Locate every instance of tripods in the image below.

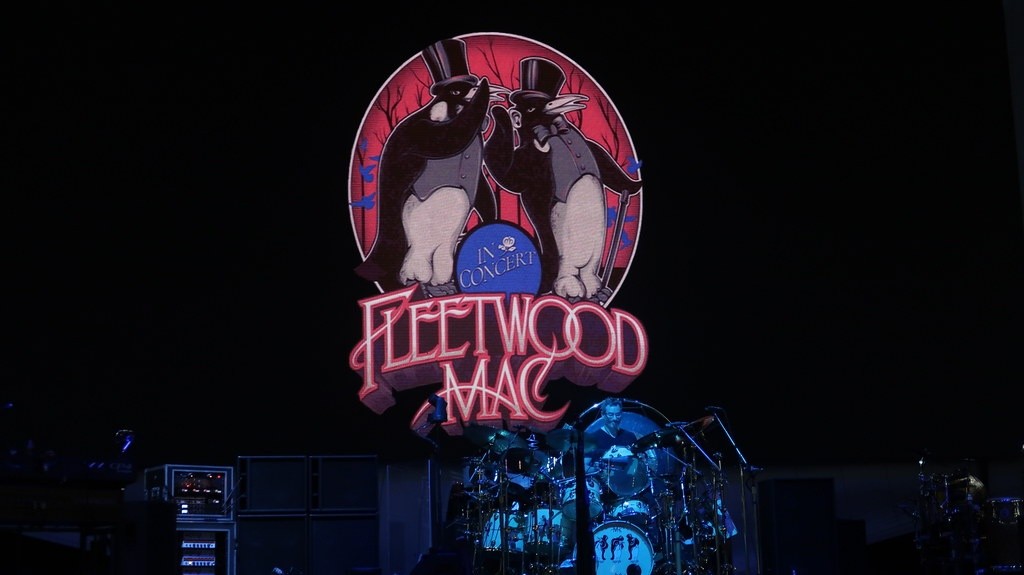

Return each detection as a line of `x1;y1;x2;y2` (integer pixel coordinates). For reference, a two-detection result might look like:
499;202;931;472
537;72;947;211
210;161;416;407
652;434;714;575
523;439;575;575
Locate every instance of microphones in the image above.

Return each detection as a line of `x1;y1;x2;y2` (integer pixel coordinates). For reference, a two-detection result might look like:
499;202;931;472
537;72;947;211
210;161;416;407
705;406;723;410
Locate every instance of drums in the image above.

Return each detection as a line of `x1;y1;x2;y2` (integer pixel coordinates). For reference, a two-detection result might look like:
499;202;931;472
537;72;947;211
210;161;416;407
601;446;659;499
525;502;570;557
613;498;651;522
560;475;605;520
478;506;527;560
572;517;655;575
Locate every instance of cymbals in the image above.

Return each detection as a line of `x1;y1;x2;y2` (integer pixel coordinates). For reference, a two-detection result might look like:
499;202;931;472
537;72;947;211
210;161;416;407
631;428;678;450
677;414;715;439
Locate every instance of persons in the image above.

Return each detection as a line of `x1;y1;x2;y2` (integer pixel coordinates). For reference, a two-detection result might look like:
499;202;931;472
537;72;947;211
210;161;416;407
591;397;638;467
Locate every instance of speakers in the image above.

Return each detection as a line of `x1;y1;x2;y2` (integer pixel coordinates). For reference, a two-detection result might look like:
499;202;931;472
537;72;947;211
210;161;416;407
235;513;383;575
